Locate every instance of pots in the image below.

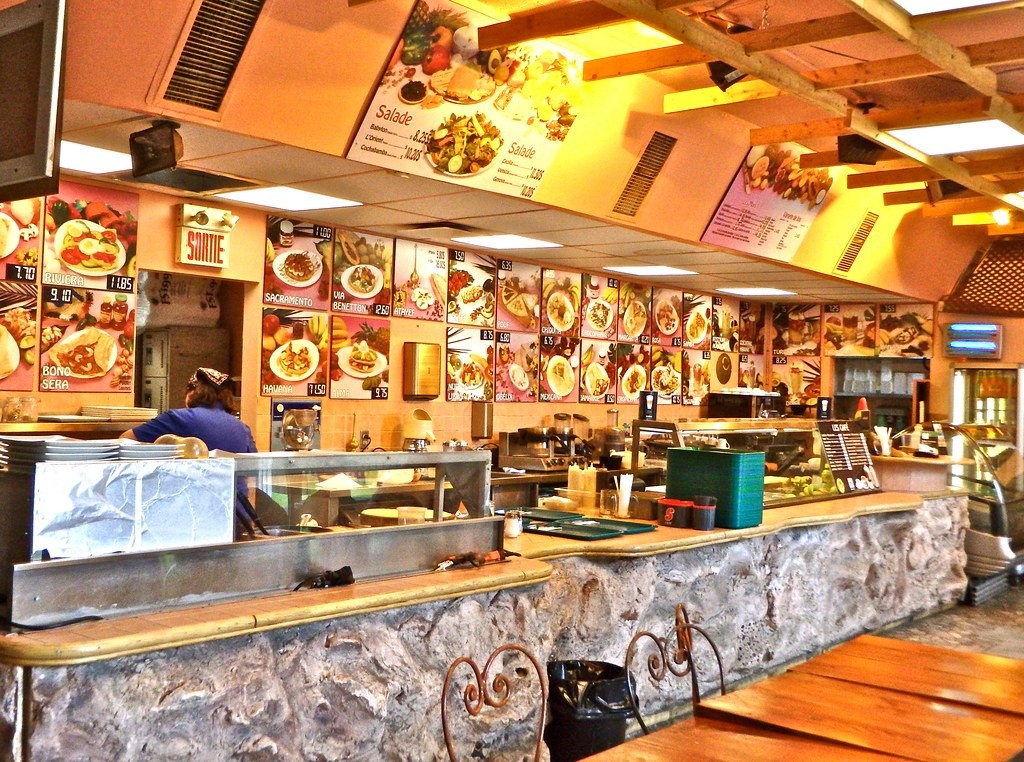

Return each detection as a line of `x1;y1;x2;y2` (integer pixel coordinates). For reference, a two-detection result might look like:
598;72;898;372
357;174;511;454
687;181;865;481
244;525;332;541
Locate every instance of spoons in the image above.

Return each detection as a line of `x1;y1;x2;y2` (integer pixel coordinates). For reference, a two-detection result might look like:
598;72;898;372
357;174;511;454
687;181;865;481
410;245;419;283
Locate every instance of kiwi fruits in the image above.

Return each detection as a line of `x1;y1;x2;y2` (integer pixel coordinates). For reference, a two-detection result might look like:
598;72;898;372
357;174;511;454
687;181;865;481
450;354;462;370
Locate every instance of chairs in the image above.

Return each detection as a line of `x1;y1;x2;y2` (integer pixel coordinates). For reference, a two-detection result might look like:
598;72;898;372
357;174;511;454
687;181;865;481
442;644;547;762
625;603;725;735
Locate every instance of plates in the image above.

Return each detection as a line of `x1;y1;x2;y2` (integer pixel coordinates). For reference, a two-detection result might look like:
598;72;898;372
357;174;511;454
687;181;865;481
686;312;708;344
546;292;575;331
624;301;647;338
54;219;127;277
269;339;320;381
337;347;387;378
655;302;679;335
397;87;428;105
621;365;647;398
0;330;20;380
586;300;614;331
272;250;323;287
547;355;575;397
0;212;20;259
509;363;530;390
459;380;482;389
430;71;497;105
585;362;609;399
425;122;502;178
0;435;186;474
651;366;681;398
341;264;383;298
62;329;118;378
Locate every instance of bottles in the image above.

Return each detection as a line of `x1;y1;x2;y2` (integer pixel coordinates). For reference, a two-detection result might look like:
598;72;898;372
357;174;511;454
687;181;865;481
607;409;619;427
568;463;597;492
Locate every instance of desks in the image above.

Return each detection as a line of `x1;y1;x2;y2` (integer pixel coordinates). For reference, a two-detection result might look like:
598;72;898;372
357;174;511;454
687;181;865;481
577;634;1024;762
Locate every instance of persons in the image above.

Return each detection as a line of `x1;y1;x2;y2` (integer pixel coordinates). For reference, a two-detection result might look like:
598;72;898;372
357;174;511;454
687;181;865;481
115;366;260;538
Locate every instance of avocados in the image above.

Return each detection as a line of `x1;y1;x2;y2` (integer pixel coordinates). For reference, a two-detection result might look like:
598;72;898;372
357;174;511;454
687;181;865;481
19;335;35;365
478;49;502;74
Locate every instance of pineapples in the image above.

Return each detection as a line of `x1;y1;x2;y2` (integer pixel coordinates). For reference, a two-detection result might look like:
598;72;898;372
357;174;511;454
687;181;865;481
402;0;431;37
349;321;390;354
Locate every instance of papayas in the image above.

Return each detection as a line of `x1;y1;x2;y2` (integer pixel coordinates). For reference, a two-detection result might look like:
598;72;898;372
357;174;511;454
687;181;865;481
339;234;361;266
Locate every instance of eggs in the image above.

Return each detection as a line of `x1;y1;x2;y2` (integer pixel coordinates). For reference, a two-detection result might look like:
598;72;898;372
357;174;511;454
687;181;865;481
80;238;99;255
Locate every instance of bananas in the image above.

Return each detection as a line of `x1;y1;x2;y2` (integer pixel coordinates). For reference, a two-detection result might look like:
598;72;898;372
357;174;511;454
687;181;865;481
603;282;632;308
308;314;352;350
542;278;581;312
470;354;488;369
581;343;594;365
266;238;275;265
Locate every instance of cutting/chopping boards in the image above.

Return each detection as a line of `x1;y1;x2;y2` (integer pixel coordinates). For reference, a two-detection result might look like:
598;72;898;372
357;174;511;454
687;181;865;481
361;507;456;527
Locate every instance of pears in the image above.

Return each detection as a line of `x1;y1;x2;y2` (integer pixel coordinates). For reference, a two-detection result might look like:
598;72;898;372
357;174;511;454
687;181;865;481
10;199;34;229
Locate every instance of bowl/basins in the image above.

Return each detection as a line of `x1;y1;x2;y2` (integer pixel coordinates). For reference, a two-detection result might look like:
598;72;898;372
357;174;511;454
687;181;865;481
790;405;807;415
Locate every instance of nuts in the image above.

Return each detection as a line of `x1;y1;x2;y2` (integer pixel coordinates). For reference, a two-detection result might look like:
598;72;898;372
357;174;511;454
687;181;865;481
109;349;133;386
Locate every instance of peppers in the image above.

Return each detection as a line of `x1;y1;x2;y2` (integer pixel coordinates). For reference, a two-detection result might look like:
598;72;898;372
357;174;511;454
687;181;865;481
401;16;453;75
120;334;133;354
76;313;97;331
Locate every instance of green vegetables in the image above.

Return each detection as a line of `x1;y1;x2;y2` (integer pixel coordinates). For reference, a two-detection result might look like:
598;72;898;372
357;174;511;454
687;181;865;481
424;113;504;174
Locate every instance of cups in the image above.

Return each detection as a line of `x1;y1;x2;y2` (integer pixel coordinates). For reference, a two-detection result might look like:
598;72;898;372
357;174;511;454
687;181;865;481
614;495;638;518
599;490;619;515
693;435;730;449
872;437;893;456
573;414;595;443
397;507;426;526
555;413;571;434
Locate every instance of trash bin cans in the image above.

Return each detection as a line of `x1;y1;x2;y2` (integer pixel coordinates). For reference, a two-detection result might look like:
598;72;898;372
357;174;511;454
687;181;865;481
547;659;639;762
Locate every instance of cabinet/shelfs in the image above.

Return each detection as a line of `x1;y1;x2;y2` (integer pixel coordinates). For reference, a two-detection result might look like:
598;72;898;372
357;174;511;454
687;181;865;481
141;325;230;417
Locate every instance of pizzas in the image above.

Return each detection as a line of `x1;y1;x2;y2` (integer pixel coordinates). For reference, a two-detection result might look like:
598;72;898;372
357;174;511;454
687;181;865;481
501;286;539;317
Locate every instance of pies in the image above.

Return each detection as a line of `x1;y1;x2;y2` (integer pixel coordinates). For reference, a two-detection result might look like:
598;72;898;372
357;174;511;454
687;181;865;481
445;66;476;100
48;326;113;378
0;325;13;376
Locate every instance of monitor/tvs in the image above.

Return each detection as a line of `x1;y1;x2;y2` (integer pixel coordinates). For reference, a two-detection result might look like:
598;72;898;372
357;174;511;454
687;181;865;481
1;0;68;204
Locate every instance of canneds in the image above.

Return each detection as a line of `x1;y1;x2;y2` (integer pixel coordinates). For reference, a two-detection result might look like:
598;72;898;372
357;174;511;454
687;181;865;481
279;220;294;247
589;276;600;299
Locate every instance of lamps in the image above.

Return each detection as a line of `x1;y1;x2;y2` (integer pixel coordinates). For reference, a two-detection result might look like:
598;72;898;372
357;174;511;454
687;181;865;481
129;120;184;178
927;179;968;202
706;25;752;92
190;210;209;225
838;102;887;165
221;212;240;229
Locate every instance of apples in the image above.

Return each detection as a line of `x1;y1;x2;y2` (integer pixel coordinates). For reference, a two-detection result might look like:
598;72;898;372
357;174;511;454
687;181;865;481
522;62;567;121
262;314;288;350
